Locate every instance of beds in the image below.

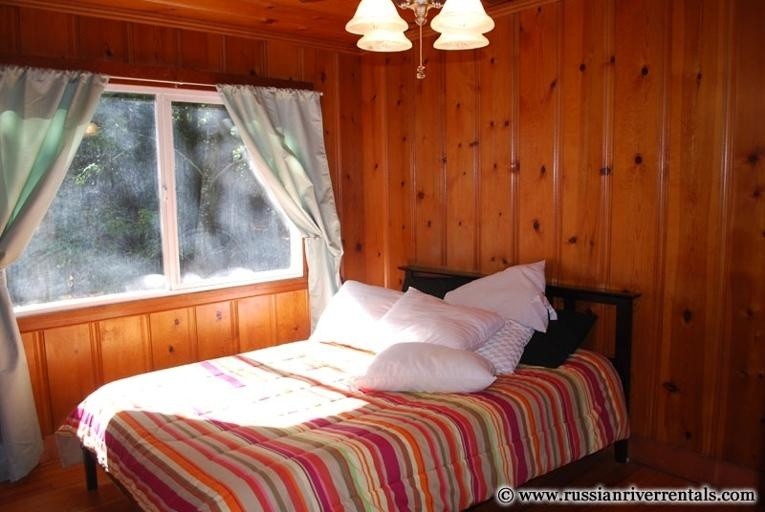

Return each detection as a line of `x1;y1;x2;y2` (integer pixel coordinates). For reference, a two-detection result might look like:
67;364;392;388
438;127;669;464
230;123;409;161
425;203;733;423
75;264;644;511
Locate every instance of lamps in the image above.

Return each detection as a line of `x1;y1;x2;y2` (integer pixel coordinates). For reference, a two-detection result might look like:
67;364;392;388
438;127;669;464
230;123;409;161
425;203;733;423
345;0;495;80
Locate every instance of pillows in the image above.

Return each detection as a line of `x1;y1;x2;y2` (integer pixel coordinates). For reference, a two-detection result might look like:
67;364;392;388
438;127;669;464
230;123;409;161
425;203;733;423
308;258;598;394
